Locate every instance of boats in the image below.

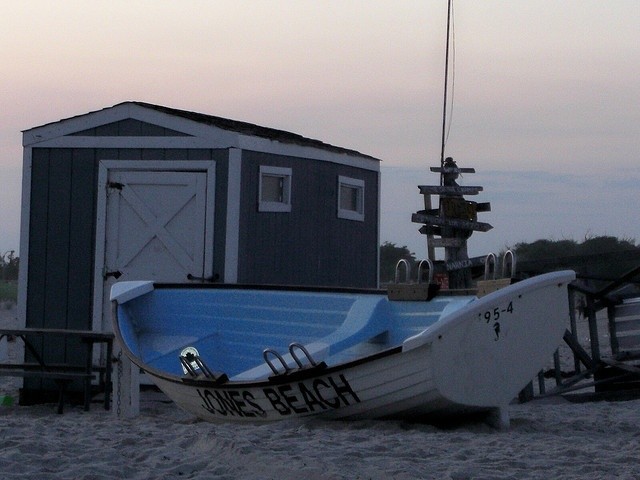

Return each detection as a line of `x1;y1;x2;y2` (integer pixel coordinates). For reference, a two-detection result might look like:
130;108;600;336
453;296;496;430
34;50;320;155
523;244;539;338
109;270;576;427
